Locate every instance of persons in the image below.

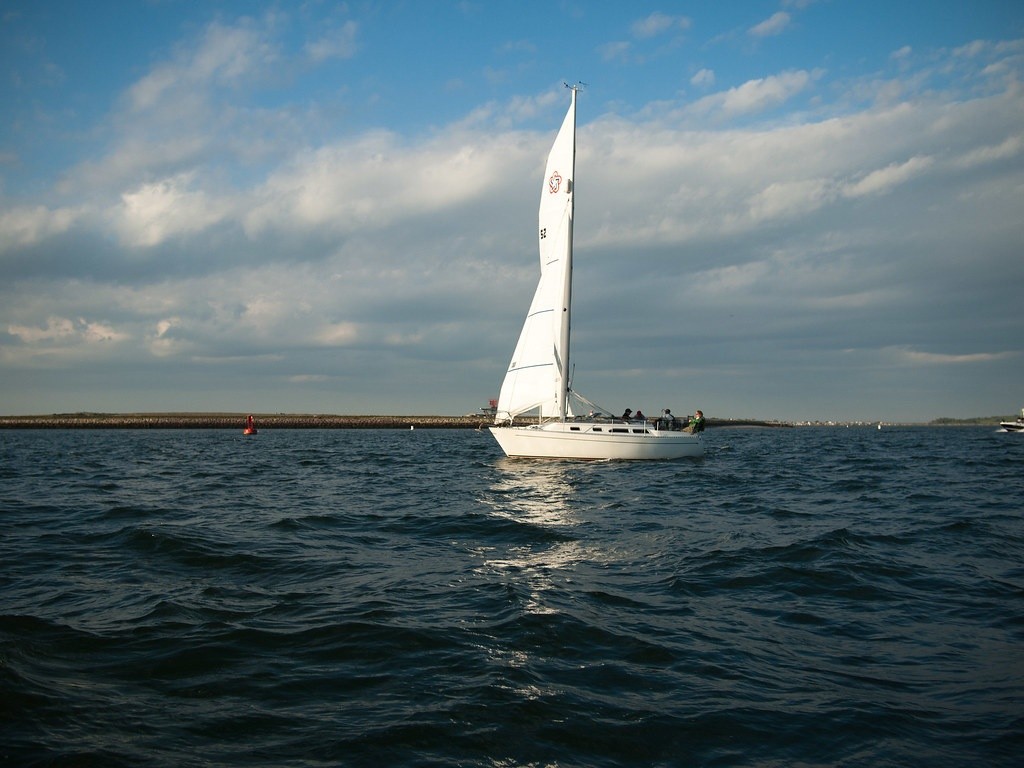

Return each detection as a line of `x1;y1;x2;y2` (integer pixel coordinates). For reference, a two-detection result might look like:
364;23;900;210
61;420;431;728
689;410;706;435
621;408;677;431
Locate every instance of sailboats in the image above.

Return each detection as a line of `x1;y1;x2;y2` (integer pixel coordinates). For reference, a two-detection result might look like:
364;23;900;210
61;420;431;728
486;78;705;461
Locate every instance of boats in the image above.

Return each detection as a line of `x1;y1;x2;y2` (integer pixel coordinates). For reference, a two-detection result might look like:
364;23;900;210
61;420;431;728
243;415;258;436
999;408;1024;434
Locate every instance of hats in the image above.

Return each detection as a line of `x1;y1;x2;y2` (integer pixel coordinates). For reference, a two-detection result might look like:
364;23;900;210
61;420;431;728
637;411;641;416
625;409;632;412
